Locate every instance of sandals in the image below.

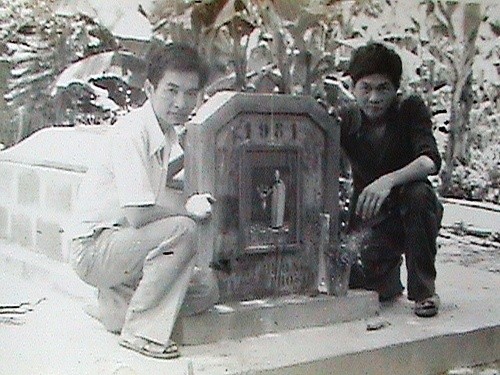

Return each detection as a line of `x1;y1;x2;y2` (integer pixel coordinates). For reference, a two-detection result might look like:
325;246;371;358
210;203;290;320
415;294;440;317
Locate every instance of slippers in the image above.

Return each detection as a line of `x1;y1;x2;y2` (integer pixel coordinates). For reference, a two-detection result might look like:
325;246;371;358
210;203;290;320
82;304;121;334
118;334;180;359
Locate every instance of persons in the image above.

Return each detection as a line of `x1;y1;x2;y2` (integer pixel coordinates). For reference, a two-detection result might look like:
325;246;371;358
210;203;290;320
315;41;445;317
60;41;220;360
